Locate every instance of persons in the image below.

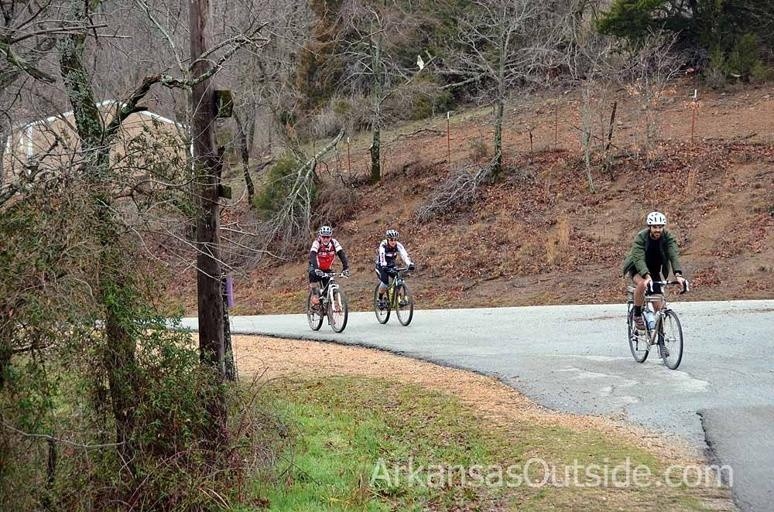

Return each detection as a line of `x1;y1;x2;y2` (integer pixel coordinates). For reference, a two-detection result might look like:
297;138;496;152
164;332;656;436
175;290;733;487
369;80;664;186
374;229;414;309
307;225;349;326
622;211;690;342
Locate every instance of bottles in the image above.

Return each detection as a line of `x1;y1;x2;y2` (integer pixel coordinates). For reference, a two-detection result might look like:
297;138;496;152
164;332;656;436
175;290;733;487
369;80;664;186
646;309;656;329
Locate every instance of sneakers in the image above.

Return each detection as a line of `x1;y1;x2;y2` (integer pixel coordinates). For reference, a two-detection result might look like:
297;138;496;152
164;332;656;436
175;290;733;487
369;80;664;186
632;314;646;330
310;294;321;305
658;345;671;359
376;296;385;311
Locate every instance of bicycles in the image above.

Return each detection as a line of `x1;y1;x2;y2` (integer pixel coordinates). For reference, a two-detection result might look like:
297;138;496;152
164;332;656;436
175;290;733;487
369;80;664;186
622;277;690;371
372;260;415;326
305;268;349;333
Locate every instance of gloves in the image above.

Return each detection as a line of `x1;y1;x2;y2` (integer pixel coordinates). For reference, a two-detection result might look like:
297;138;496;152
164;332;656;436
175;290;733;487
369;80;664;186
315;268;324;277
340;269;351;277
409;264;416;271
382;266;389;273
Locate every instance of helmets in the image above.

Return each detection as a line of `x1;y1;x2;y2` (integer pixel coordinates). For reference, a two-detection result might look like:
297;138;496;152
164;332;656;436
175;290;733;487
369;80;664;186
385;229;400;241
646;211;668;227
318;225;333;237
